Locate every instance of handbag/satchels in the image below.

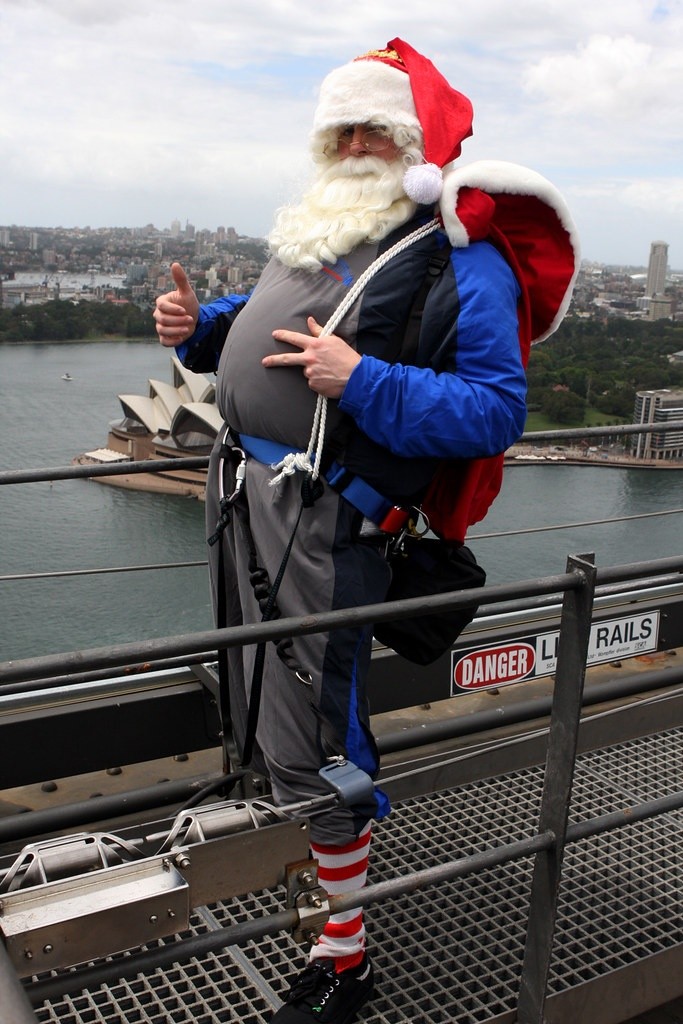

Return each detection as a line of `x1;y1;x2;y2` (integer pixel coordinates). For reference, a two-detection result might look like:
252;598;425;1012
373;536;487;667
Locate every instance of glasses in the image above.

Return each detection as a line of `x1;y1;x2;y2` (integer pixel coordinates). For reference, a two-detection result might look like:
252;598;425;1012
322;130;391;160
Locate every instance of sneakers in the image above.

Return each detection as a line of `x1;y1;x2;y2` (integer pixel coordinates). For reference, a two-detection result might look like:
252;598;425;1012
269;951;373;1023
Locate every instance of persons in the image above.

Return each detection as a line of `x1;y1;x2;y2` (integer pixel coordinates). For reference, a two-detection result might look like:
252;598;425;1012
152;37;528;1024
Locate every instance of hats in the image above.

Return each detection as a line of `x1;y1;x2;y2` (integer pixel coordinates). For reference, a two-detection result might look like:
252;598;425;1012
313;37;474;204
